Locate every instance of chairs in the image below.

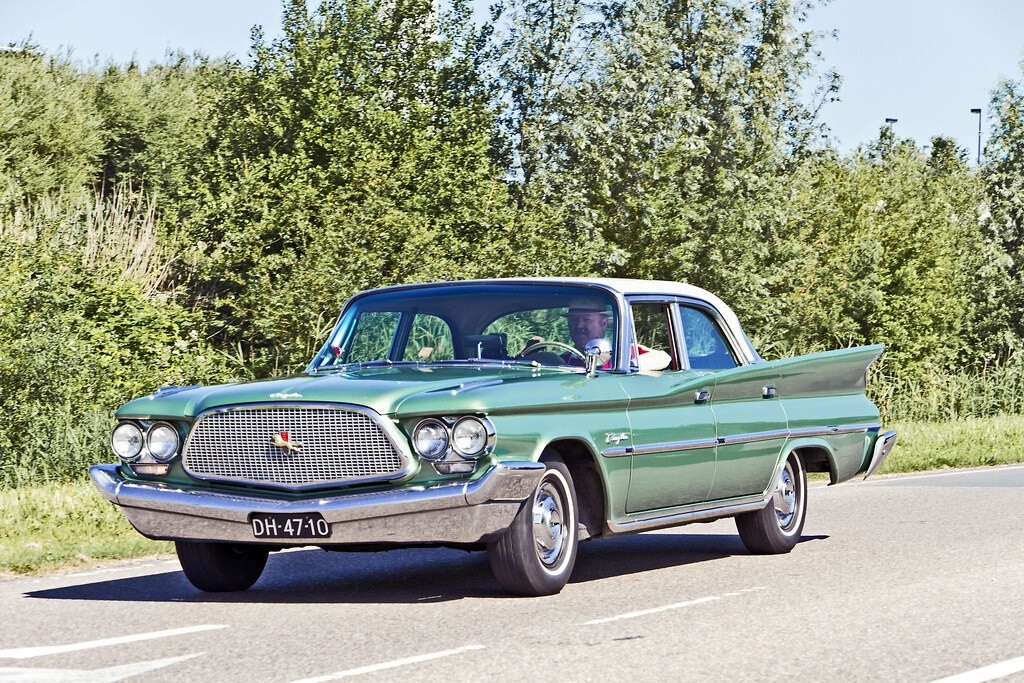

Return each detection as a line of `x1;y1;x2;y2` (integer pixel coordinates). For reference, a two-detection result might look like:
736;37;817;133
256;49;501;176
462;333;507;360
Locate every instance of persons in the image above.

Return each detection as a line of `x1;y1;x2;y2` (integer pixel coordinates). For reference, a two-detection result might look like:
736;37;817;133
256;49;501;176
559;294;672;371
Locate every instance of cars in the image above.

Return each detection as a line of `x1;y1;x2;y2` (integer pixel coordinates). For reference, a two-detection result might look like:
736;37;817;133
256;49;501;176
87;275;900;598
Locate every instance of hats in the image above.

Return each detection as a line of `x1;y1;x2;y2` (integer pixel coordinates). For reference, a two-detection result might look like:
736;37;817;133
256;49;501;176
560;295;613;317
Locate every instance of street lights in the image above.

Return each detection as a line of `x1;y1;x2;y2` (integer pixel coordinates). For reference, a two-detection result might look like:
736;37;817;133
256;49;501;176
884;117;898;156
970;107;982;171
